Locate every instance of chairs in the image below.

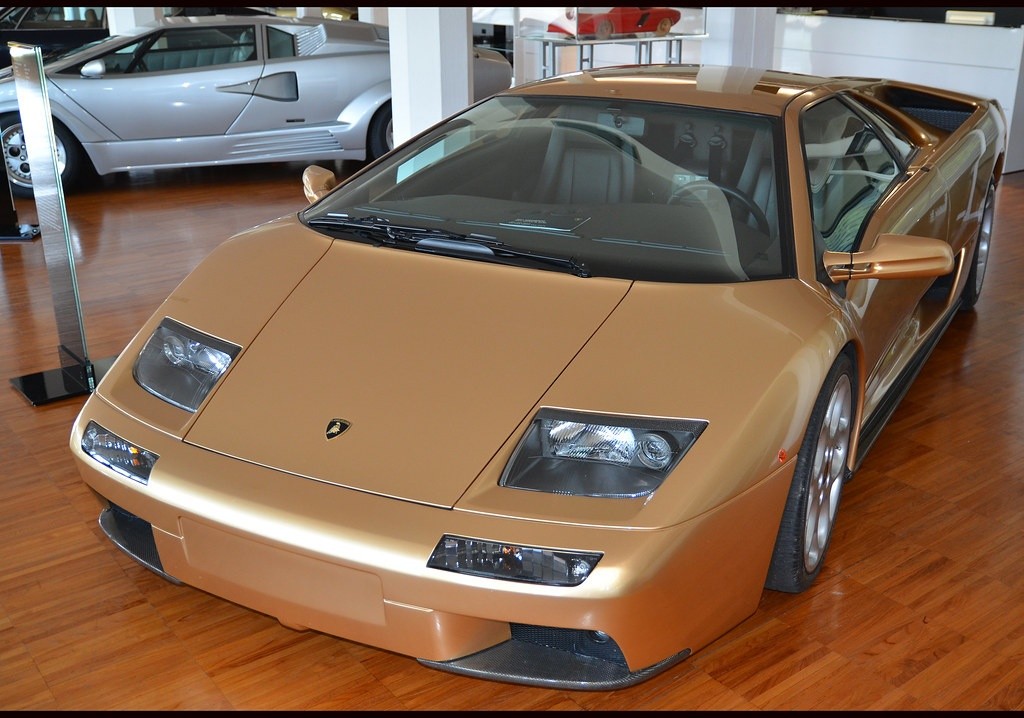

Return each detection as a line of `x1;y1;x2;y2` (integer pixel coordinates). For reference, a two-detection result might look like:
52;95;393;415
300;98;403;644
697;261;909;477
741;113;853;246
523;102;642;207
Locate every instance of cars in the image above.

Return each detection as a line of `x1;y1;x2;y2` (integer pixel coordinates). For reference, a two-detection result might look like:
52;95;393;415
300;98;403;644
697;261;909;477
0;6;359;73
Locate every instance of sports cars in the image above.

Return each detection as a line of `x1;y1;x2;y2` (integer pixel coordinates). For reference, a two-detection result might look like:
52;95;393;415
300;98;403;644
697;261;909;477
0;15;513;201
544;7;684;41
66;62;1009;692
